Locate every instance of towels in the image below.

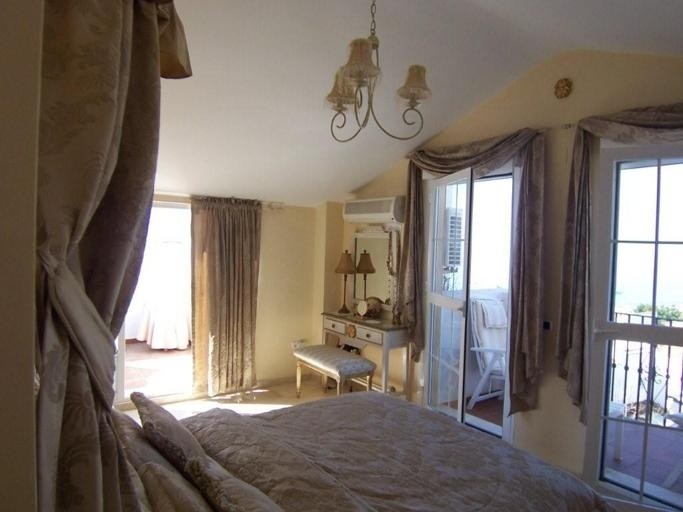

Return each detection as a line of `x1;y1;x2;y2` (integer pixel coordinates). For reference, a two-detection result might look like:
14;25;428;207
475;297;507;328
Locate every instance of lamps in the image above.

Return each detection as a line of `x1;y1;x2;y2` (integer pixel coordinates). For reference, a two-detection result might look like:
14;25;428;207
332;250;356;314
315;0;431;145
354;250;376;300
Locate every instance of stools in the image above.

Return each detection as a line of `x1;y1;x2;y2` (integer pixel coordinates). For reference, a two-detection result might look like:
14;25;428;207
661;413;683;490
293;343;377;395
607;399;625;463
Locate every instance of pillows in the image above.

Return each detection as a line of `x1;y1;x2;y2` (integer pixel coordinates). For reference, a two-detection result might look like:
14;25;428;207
109;392;284;511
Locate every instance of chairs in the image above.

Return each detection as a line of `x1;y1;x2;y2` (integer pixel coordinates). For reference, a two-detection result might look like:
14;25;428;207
468;295;506;411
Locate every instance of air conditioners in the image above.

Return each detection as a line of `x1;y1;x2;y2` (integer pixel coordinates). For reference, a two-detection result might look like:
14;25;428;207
443;207;462;274
341;196;405;225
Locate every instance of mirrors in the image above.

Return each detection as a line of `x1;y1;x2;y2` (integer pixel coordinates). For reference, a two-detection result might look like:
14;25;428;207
350;225;400;313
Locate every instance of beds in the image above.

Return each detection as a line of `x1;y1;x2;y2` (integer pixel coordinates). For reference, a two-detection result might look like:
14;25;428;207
108;383;617;511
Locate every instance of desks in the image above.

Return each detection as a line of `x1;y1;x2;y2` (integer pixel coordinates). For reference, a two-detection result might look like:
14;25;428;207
323;310;410;402
135;309;192;352
